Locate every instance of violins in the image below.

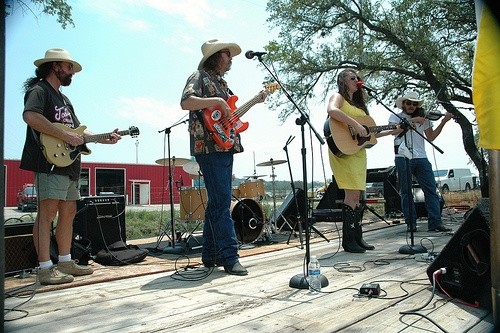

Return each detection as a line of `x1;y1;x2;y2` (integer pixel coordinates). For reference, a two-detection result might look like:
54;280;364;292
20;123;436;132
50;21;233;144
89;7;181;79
413;108;460;124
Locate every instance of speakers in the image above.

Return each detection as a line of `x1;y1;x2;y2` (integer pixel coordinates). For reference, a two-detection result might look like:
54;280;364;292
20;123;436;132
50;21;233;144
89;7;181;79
3;222;40;276
271;187;315;231
410;183;445;217
75;195;125;253
312;177;345;222
425;197;495;311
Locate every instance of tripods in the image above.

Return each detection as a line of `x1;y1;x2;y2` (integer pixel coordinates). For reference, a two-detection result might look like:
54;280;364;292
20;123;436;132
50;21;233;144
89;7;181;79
283;136;329;249
269;164;297;237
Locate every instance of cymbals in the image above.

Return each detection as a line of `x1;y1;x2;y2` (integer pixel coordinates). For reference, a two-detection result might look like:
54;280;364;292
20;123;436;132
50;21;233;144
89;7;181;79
155;158;191;166
243;175;268;177
183;161;202;175
256;160;288;166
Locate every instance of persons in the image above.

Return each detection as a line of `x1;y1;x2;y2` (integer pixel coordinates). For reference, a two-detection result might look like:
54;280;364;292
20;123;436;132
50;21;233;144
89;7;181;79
389;92;454;231
180;39;268;275
19;48;122;284
327;70;404;253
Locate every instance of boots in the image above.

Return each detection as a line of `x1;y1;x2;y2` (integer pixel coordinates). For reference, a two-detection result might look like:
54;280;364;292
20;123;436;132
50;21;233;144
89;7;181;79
354;204;374;250
341;203;366;253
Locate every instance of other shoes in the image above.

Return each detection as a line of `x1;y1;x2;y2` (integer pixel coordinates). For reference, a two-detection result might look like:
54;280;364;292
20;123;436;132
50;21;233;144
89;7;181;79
437;224;452;231
39;266;74;284
58;259;93;275
407;224;417;231
203;258;224;266
224;262;248;276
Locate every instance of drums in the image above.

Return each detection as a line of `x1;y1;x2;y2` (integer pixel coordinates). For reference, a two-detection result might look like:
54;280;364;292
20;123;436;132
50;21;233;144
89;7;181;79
240;181;265;198
230;197;264;245
230;188;240;200
179;187;209;221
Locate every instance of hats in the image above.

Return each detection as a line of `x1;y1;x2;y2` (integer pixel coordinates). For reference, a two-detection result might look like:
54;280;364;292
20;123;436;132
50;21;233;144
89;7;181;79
197;39;241;70
34;49;82;74
396;92;424;110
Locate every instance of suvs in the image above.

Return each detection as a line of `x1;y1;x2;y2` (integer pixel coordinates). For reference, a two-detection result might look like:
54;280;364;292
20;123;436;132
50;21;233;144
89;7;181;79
16;183;40;213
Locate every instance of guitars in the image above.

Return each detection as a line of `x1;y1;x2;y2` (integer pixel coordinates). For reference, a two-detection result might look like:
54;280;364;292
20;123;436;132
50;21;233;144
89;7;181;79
324;115;417;159
202;79;281;150
40;123;139;167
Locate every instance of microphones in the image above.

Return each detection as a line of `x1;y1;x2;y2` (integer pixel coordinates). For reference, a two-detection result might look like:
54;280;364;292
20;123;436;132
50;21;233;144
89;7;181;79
244;51;267;58
356;82;376;92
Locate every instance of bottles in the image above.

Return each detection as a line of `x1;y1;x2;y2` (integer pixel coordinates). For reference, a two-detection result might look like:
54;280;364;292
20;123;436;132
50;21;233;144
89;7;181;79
308;256;321;294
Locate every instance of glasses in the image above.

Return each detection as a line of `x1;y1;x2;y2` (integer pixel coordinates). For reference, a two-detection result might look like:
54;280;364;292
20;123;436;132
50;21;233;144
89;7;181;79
406;102;417;106
345;77;360;81
55;62;73;71
219;51;230;57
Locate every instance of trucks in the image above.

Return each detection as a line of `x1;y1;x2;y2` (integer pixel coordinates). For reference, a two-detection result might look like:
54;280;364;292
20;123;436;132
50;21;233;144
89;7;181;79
365;168;389;198
434;168;474;192
